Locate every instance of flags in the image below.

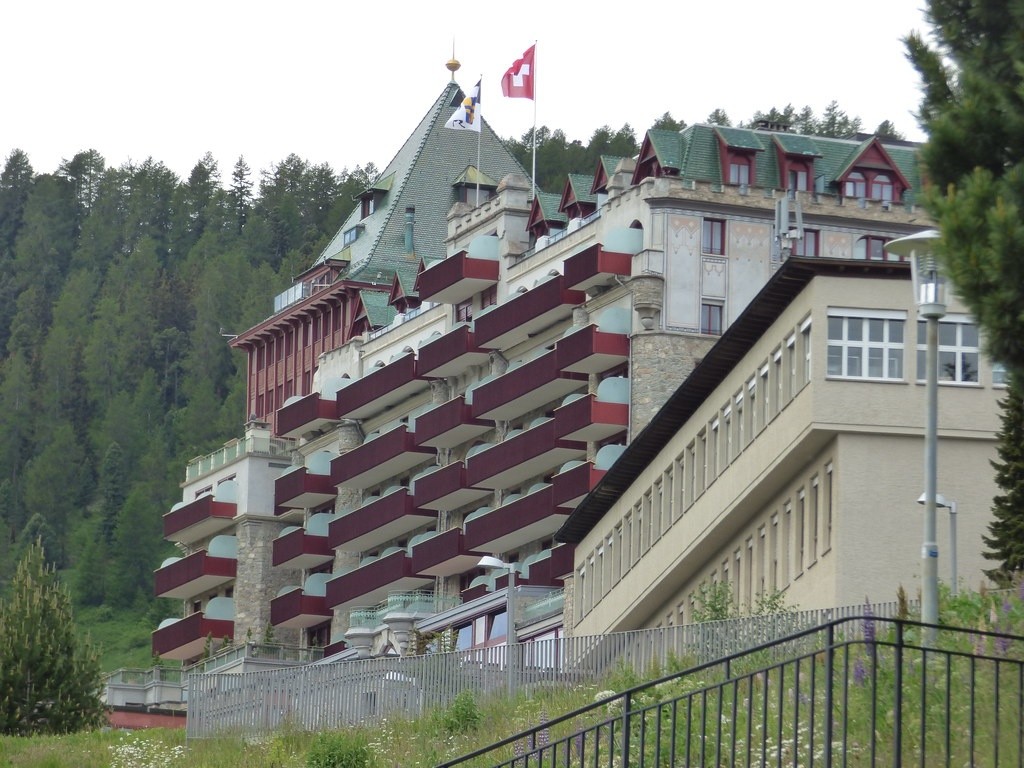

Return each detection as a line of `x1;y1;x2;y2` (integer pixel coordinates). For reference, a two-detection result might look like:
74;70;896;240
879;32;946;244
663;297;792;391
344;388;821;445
501;44;536;103
443;80;482;133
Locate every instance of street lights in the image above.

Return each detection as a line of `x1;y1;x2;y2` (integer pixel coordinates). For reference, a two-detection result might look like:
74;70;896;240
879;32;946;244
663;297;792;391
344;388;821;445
916;493;960;595
882;229;951;646
478;555;517;680
